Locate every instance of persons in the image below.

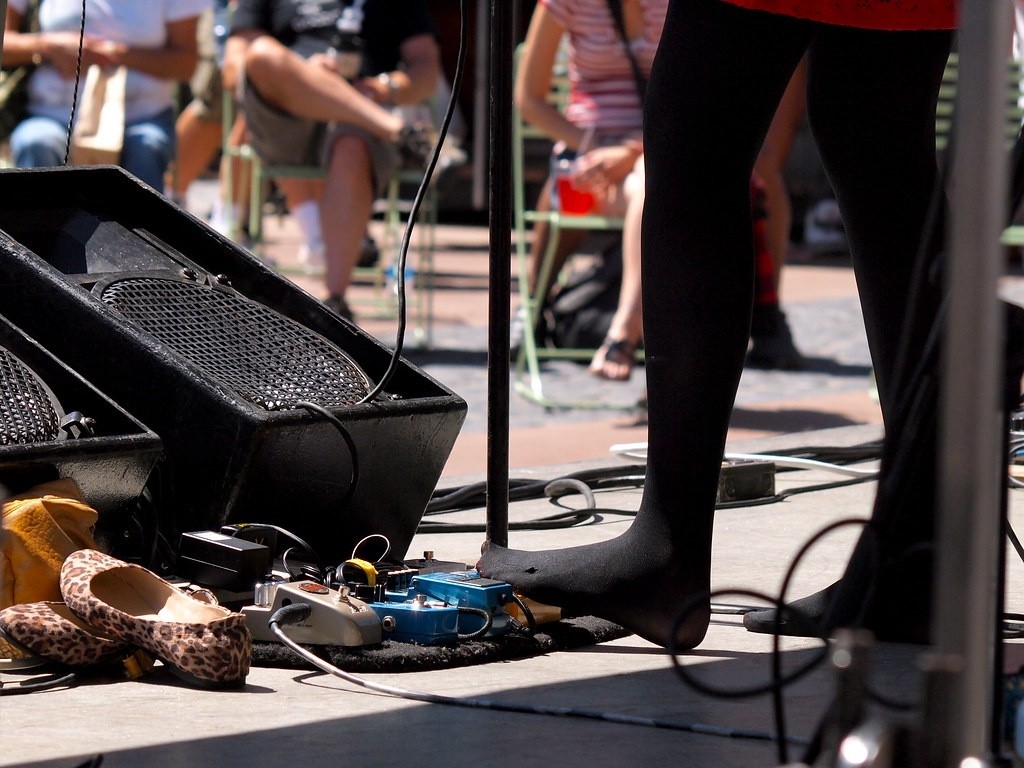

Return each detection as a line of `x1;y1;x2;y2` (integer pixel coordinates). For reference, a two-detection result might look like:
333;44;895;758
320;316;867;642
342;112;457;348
0;0;809;380
476;0;962;652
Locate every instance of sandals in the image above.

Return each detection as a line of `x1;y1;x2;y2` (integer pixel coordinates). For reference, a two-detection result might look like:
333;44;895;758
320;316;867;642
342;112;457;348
590;334;635;382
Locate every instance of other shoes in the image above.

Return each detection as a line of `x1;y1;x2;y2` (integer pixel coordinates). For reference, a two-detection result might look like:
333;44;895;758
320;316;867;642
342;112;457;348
354;233;380;268
395;100;439;167
305;246;327;278
749;313;798;366
326;295;355;326
1;585;216;674
59;547;253;692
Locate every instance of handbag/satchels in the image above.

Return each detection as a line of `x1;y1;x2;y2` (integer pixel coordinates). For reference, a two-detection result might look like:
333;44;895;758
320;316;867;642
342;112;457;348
66;61;132;170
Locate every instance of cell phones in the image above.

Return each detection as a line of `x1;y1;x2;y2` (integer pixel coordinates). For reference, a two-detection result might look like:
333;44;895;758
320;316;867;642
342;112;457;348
328;49;362;77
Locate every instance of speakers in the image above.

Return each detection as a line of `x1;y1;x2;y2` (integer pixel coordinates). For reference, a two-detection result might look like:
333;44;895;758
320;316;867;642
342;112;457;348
0;165;468;573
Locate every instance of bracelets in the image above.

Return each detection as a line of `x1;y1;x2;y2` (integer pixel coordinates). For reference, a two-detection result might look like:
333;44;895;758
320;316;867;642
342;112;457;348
377;72;399;101
33;34;43;67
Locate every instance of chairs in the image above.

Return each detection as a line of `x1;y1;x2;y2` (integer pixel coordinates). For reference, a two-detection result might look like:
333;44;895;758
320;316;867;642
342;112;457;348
512;42;647;412
220;88;436;358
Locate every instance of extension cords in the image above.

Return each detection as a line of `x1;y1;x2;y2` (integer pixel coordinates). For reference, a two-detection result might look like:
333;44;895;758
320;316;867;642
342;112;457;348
166;569;290;611
